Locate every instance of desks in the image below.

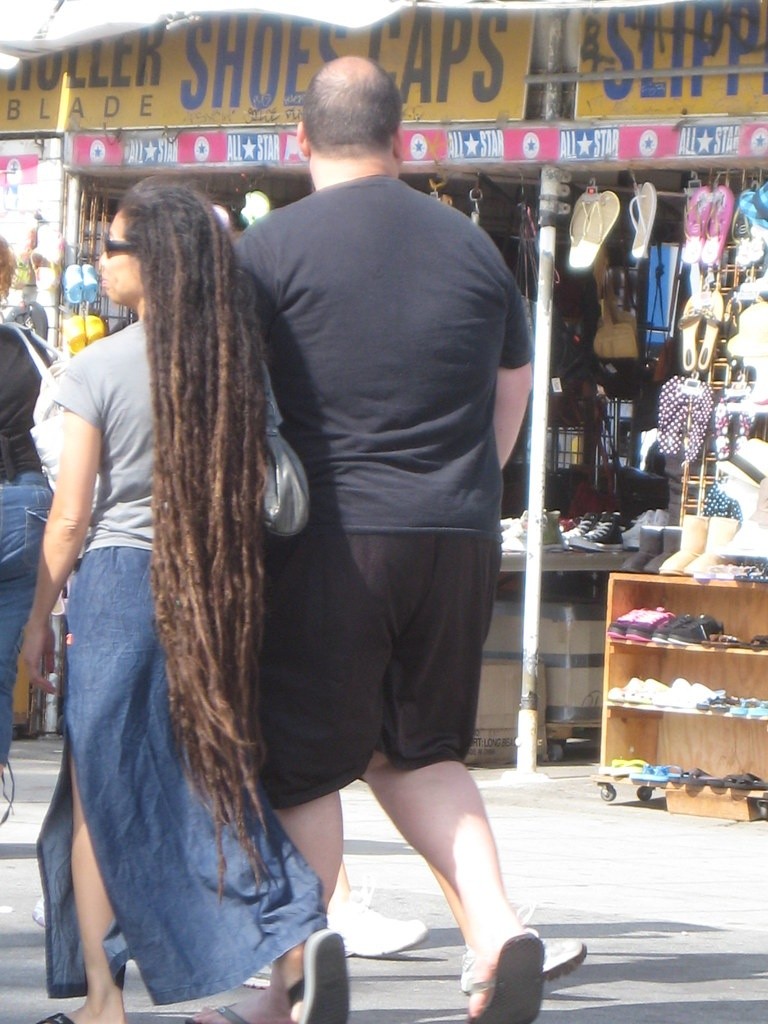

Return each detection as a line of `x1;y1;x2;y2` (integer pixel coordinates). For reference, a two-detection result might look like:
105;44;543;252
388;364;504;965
500;552;639;572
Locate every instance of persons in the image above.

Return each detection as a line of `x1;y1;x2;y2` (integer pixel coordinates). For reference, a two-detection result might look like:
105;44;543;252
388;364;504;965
0;234;64;826
236;56;585;1024
20;177;352;1024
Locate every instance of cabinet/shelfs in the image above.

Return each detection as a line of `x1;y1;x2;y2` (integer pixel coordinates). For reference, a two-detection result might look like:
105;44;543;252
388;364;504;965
591;573;768;801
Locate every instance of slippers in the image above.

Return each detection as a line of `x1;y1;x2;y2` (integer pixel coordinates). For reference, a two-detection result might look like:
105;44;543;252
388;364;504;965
61;264;99;304
700;633;768;651
4;302;48;341
63;315;104;354
35;1012;74;1024
656;184;768;462
605;677;768;718
286;929;348;1024
569;190;621;269
464;931;545;1024
184;1002;253;1024
658;514;738;576
599;758;767;791
629;182;657;258
10;225;64;292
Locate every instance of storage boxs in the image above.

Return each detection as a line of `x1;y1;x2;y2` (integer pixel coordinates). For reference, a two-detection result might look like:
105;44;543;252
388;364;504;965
464;592;606;764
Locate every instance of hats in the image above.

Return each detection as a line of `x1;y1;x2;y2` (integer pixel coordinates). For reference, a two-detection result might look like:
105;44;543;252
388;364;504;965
738;182;768;229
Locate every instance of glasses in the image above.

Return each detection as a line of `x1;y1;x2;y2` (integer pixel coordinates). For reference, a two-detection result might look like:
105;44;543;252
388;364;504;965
104;234;139;259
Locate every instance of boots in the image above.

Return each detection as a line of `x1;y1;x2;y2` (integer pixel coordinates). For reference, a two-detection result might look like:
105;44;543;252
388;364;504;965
617;526;683;576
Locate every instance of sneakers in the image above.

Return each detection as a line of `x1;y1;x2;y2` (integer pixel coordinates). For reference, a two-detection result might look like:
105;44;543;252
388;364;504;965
501;508;670;554
622;607;677;641
608;606;646;639
652;612;694;645
667;613;726;648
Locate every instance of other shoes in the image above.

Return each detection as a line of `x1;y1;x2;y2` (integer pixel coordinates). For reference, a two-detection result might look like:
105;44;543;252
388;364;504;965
458;934;588;995
326;886;429;958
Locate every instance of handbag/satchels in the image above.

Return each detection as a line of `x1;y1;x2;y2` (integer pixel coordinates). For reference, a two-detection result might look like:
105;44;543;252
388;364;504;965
254;358;310;537
14;325;100;518
593;308;676;431
